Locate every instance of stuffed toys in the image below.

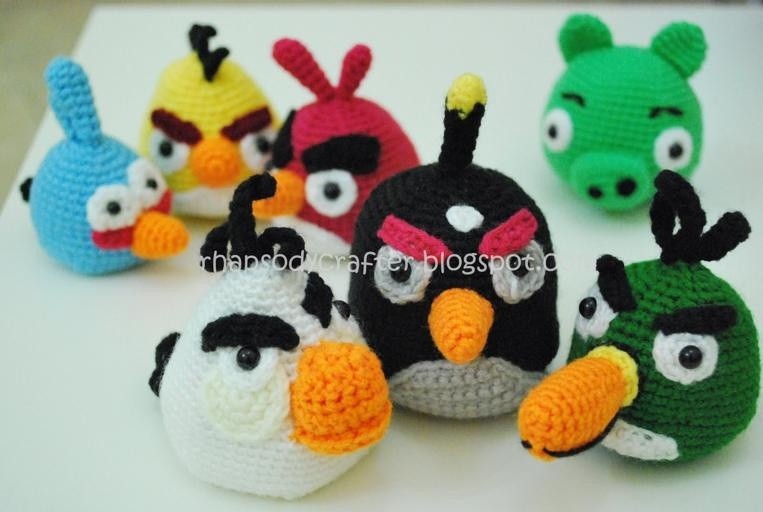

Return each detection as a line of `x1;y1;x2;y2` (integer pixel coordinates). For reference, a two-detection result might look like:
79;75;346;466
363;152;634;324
15;49;189;280
